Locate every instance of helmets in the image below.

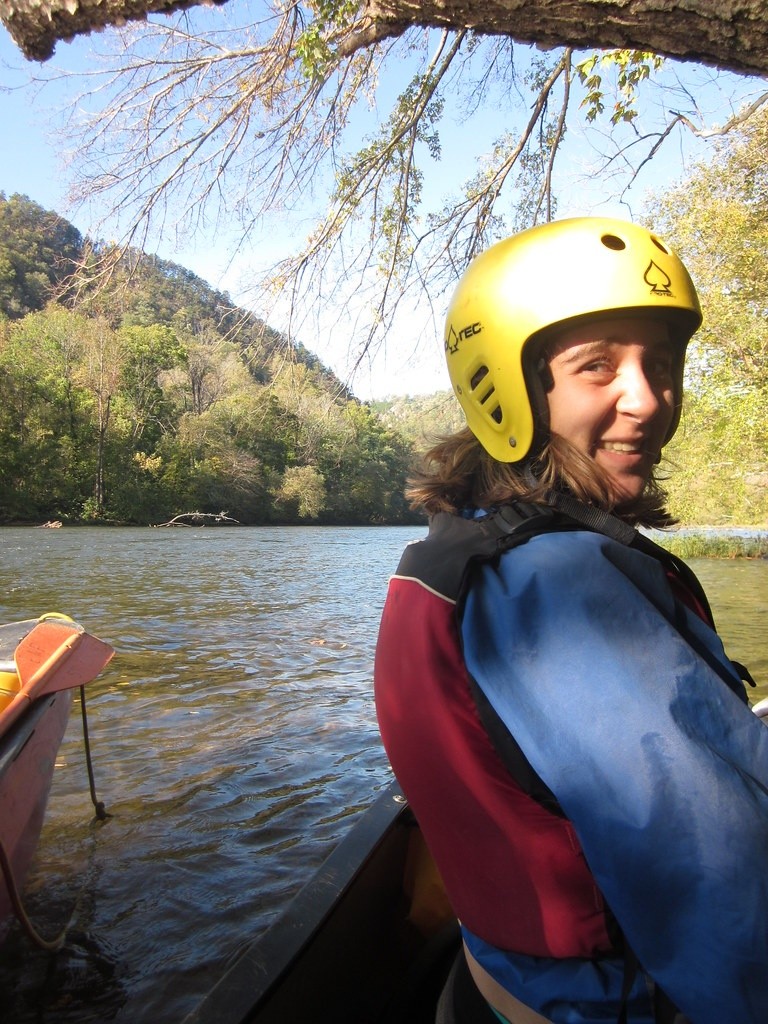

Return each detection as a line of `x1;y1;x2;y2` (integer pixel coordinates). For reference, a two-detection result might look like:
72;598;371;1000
444;217;703;462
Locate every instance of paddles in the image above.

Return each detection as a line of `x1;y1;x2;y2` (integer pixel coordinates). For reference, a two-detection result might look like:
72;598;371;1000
1;619;117;728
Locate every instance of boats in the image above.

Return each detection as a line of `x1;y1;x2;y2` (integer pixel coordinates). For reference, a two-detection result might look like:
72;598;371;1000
0;611;116;953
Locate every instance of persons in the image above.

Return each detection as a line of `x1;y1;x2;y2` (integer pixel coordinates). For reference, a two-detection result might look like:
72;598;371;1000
373;215;768;1024
